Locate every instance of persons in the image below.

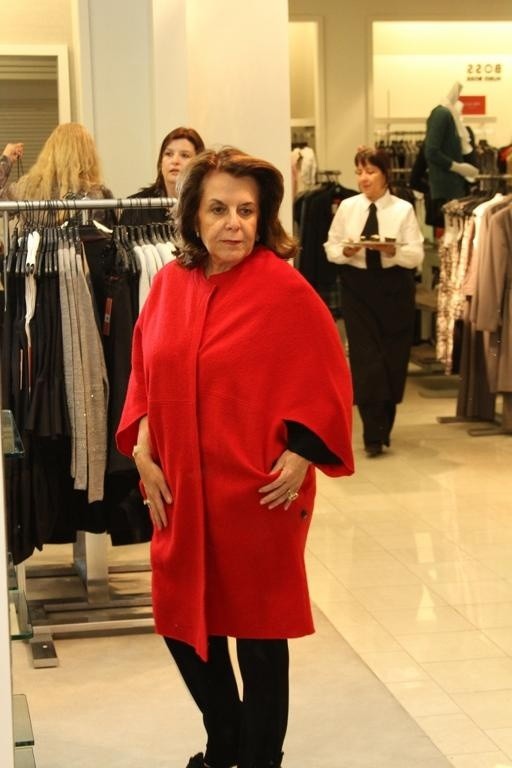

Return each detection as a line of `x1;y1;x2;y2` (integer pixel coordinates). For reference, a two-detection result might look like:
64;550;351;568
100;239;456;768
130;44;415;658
0;122;119;231
120;127;204;224
425;80;482;200
115;144;356;767
324;147;425;459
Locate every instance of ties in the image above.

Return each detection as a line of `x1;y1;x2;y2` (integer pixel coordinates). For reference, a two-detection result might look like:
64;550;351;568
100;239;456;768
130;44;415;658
361;202;383;284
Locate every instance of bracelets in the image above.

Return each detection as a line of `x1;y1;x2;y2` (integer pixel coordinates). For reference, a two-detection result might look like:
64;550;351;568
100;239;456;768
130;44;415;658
131;443;149;457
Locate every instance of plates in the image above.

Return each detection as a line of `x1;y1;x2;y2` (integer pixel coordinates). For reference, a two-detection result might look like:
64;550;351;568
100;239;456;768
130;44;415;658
341;243;410;247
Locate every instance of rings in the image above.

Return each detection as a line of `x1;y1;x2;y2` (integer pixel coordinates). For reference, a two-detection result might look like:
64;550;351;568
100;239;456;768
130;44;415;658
287;489;299;501
141;497;151;506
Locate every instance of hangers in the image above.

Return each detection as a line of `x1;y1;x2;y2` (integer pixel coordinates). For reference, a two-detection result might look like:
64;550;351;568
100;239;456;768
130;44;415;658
324;170;341;186
5;197;181;279
440;171;506;216
